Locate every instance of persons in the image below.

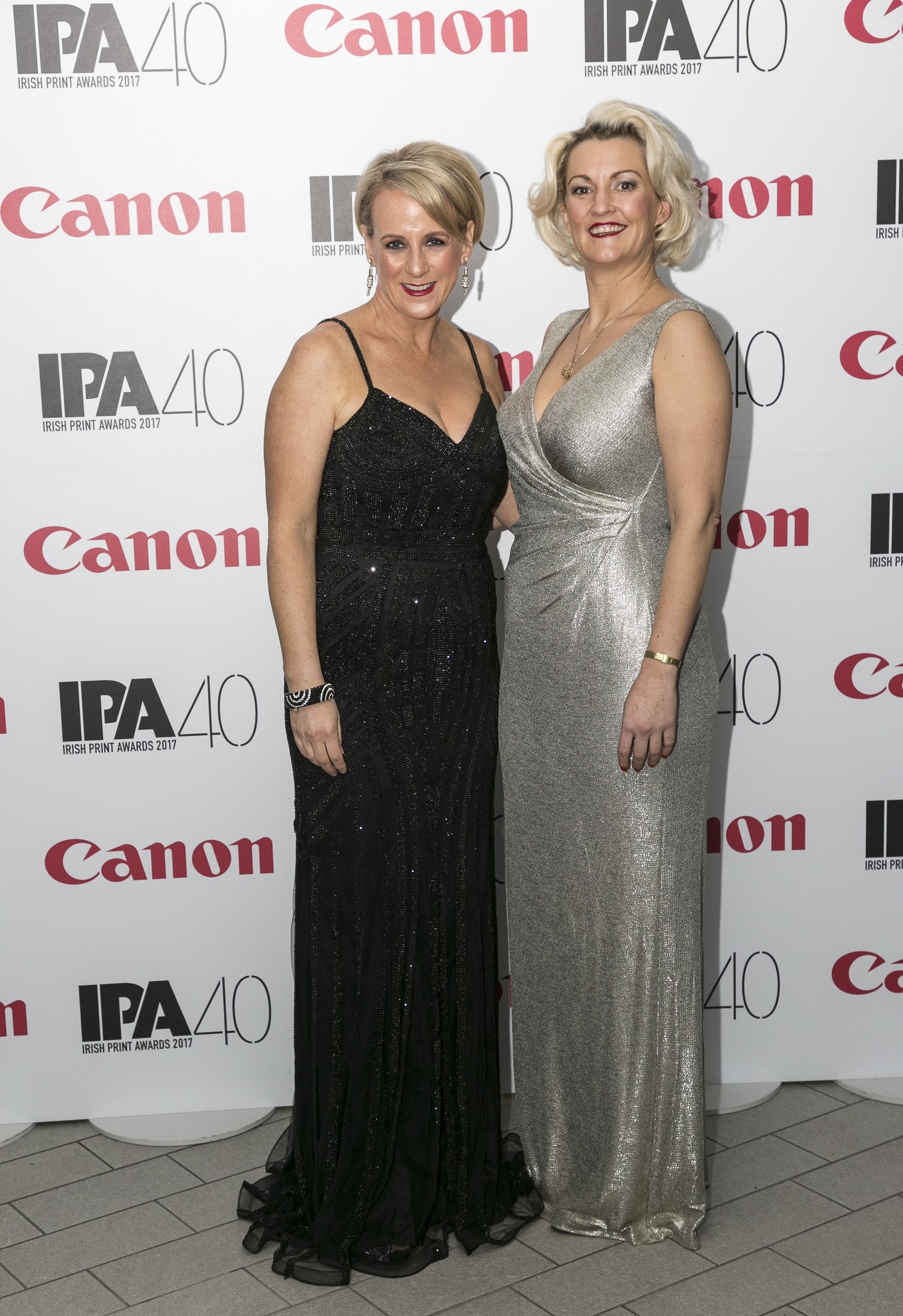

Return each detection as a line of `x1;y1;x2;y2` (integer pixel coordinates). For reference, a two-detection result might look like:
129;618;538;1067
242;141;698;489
492;102;736;1258
242;142;547;1291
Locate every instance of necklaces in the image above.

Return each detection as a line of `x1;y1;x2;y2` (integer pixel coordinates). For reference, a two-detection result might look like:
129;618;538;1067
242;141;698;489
560;274;662;376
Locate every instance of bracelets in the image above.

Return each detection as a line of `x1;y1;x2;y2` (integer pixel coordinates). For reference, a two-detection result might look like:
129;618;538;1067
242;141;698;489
642;649;682;672
285;683;337;713
